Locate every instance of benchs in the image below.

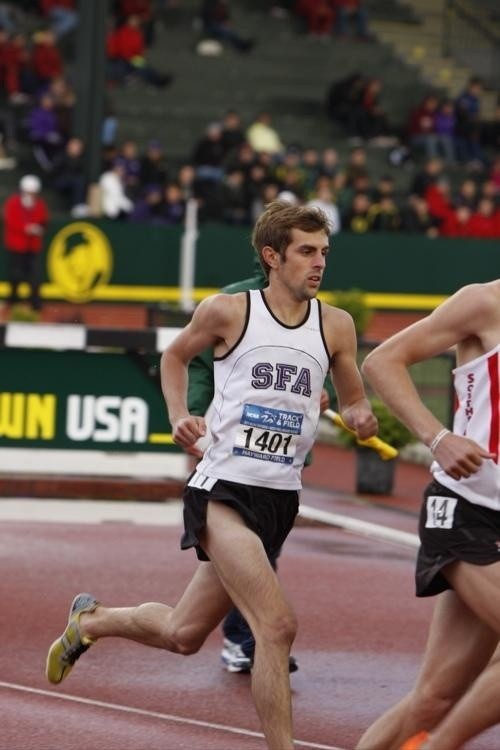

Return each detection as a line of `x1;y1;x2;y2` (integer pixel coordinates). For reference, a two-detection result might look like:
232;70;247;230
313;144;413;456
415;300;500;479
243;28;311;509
2;2;499;239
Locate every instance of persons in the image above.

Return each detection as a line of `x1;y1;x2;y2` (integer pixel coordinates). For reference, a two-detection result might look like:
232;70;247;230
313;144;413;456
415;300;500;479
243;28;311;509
181;266;334;675
43;200;380;750
349;272;500;750
1;0;500;317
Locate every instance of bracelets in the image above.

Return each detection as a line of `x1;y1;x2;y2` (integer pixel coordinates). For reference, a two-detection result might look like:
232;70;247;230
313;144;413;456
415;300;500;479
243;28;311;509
429;429;456;454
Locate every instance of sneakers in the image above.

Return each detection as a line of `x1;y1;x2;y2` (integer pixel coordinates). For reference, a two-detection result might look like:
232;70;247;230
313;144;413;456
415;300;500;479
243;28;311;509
46;593;99;682
221;637;252;673
